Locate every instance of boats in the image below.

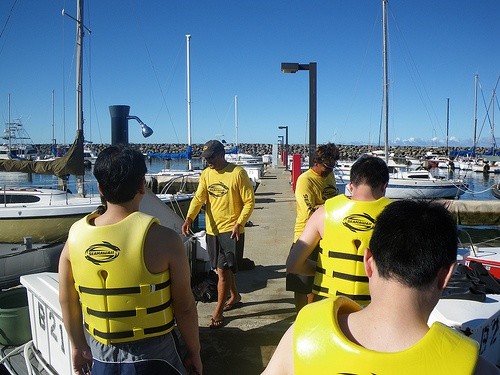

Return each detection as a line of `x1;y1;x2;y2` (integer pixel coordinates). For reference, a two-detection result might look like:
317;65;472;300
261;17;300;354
0;1;500;375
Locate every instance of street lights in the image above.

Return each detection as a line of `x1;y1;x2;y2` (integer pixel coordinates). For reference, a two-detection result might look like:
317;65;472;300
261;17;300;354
281;62;318;168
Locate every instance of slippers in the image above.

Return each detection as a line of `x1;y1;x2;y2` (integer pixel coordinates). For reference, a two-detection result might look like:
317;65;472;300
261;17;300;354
209;316;224;329
222;301;243;312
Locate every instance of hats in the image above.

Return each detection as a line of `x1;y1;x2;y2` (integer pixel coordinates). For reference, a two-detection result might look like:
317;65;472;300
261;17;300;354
199;139;226;158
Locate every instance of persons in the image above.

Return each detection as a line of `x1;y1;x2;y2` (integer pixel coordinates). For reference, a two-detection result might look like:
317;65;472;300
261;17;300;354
285;156;393;308
292;143;340;316
181;140;255;329
57;144;203;375
261;191;500;375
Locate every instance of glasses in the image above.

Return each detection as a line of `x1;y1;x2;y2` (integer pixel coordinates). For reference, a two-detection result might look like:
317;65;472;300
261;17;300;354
321;159;338;169
